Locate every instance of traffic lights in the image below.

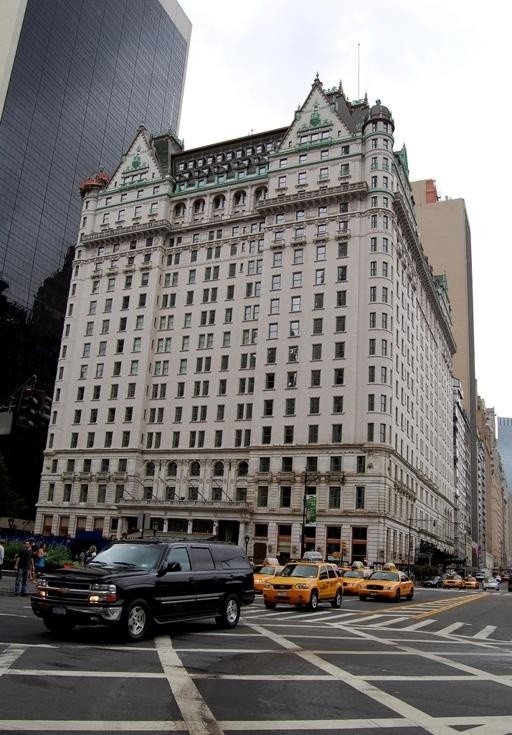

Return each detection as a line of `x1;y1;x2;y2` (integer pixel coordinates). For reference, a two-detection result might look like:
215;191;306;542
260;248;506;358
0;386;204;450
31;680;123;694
15;384;45;430
37;392;53;428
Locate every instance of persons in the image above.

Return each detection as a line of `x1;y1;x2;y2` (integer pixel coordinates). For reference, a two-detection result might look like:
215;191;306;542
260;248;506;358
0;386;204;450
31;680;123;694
249;558;254;566
0;536;98;597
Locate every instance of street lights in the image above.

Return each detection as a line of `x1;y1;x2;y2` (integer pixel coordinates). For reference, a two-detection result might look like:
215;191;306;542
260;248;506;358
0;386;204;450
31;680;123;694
244;535;250;555
464;528;468;577
406;519;436;578
152;521;159;537
299;468;348;559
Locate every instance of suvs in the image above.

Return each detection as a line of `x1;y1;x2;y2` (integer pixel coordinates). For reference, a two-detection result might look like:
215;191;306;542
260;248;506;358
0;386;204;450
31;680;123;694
27;534;257;642
508;574;512;591
260;551;346;612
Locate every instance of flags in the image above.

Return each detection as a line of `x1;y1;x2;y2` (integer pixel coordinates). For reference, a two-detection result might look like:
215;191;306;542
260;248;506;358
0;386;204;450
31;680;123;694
304;494;317;527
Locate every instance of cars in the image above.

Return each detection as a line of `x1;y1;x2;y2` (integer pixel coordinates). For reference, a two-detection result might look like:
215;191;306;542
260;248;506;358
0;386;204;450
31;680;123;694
337;559;377;595
462;574;480;589
472;568;512;583
482;578;500;591
441;571;464;589
358;562;414;603
245;557;287;593
423;574;441;588
